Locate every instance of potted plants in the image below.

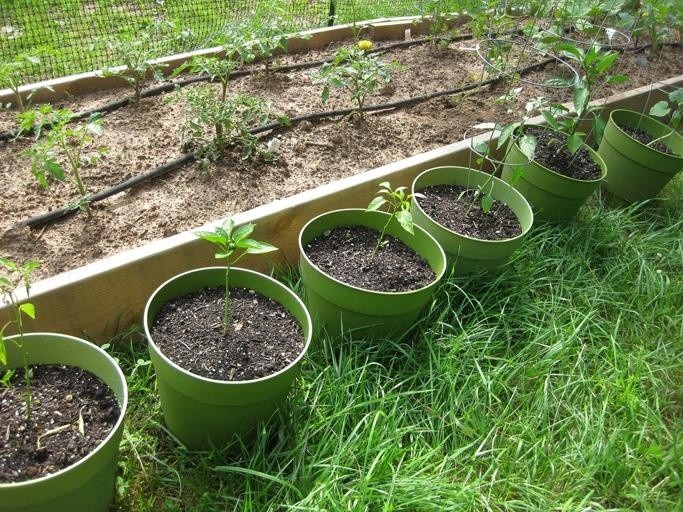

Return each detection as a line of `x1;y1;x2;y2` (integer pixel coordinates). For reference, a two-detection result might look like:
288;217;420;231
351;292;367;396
500;43;628;224
143;216;312;450
410;87;571;278
299;180;446;361
594;86;683;203
0;252;128;512
